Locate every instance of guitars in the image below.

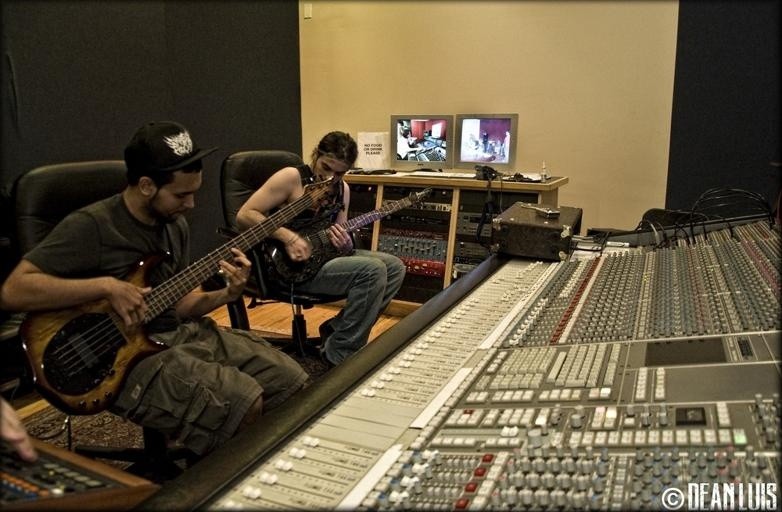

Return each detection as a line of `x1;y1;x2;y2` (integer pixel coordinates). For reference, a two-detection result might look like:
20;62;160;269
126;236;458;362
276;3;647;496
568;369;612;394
256;185;435;284
17;172;336;414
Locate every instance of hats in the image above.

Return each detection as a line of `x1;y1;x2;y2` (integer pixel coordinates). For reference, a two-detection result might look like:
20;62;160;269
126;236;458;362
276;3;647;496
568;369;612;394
124;120;219;174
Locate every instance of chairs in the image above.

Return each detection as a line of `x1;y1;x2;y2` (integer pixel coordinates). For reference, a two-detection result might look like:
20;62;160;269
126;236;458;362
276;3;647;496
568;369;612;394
219;149;328;359
10;161;196;482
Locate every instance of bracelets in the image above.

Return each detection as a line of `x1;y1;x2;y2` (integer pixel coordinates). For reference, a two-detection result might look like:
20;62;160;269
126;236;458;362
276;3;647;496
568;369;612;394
285;232;302;248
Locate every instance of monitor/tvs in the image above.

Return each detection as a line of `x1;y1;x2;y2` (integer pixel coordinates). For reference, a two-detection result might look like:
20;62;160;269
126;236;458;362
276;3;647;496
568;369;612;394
454;112;520;173
389;113;453;172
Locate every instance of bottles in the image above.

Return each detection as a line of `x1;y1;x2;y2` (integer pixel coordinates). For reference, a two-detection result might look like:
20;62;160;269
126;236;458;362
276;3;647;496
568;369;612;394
541;160;547;183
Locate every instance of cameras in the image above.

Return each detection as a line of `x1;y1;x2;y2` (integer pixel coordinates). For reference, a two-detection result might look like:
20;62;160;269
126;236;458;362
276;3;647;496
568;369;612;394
475;163;498;181
536;206;561;219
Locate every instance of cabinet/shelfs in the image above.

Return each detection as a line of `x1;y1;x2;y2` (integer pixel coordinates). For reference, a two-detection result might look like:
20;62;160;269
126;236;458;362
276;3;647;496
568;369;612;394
343;171;568;318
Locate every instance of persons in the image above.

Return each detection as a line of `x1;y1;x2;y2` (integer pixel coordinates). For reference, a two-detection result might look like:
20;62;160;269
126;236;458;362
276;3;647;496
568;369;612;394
1;119;311;460
0;389;41;466
397;128;419;159
235;132;405;377
502;130;510;163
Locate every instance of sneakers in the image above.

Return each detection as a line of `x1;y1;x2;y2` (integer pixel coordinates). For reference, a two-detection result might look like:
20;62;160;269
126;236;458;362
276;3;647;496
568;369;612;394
319;318;335;365
